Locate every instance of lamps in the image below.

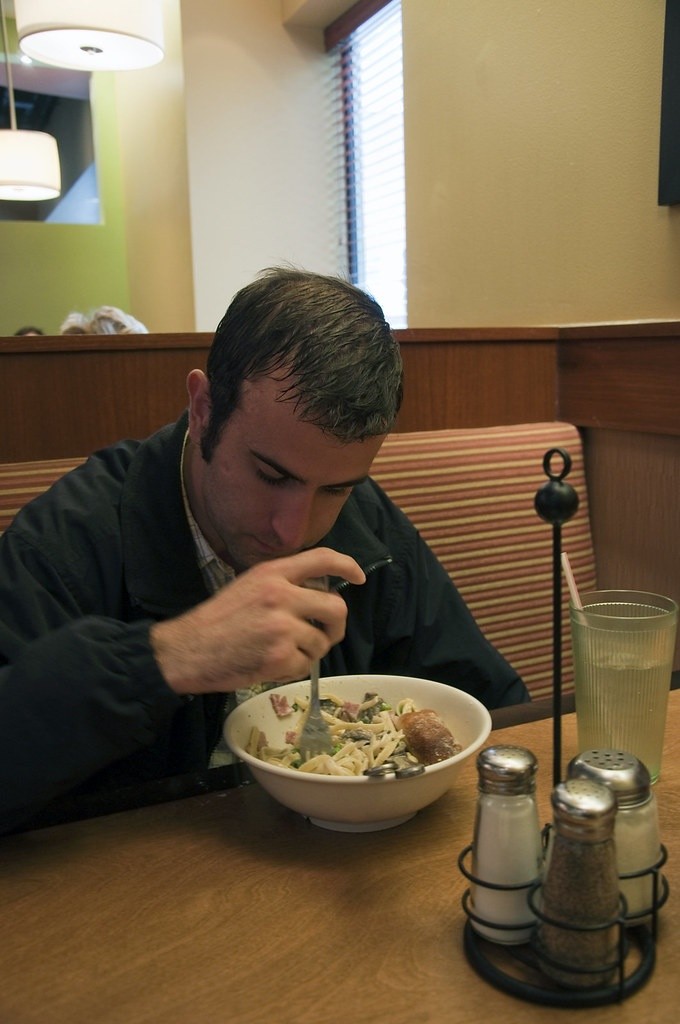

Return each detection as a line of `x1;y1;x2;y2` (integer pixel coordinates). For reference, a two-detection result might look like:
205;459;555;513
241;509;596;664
0;0;62;202
13;0;165;70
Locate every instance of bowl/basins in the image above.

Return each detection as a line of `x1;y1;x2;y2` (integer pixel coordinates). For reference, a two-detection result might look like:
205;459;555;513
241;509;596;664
224;674;492;833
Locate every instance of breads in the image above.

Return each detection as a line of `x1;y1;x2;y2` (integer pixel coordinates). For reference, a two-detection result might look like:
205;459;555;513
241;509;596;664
397;709;461;767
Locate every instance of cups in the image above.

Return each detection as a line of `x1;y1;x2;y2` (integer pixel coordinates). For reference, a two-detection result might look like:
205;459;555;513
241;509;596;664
569;590;678;786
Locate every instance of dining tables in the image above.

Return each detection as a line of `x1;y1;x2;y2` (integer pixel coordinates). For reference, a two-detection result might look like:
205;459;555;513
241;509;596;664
0;685;680;1024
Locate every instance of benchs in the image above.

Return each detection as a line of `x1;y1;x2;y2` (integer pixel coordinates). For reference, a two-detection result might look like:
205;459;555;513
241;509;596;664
0;419;600;703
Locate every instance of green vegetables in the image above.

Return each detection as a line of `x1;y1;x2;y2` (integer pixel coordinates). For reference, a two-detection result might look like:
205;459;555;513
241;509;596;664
289;692;396;767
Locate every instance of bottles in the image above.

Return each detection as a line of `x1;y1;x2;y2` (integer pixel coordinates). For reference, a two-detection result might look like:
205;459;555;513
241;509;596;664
469;745;543;943
565;750;662;924
534;781;620;992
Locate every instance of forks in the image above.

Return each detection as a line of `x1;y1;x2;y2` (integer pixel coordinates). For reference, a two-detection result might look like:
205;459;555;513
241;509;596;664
298;575;334;766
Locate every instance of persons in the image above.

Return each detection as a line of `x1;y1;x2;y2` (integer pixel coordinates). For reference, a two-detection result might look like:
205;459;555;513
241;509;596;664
0;267;534;839
60;305;150;336
11;326;45;336
